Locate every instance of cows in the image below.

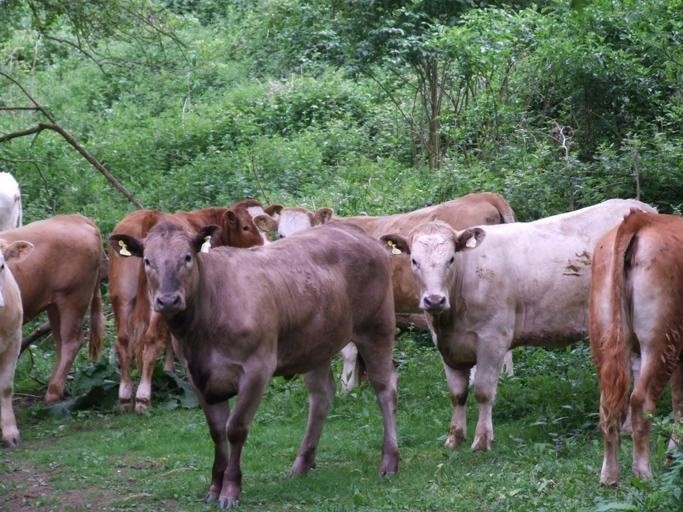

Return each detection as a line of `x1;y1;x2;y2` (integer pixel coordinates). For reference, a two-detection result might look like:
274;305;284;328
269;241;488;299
109;221;400;510
0;214;105;403
588;208;683;488
0;241;34;449
0;171;23;232
107;198;282;416
378;197;659;453
252;191;516;397
